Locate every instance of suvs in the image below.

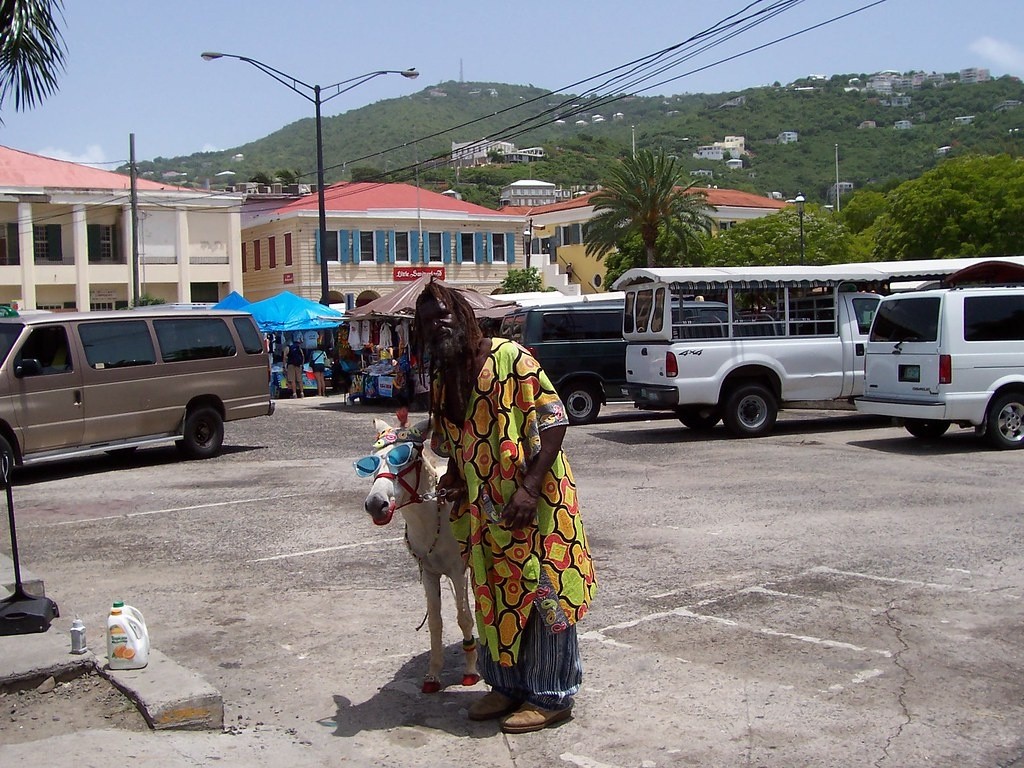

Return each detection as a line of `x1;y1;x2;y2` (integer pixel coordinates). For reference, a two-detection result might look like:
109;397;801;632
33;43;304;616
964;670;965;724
854;283;1024;450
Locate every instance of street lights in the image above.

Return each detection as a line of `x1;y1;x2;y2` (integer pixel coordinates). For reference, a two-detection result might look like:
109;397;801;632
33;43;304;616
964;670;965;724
796;192;807;265
201;51;419;351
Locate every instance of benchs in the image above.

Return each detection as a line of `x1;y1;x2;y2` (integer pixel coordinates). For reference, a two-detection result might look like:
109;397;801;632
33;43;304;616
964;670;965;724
734;315;776;336
680;317;724;338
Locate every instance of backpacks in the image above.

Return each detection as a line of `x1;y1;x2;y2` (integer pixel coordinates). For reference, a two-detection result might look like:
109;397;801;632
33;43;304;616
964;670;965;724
289;344;302;365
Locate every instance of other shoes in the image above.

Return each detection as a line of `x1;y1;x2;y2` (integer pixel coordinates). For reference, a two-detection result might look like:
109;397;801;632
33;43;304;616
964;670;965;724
300;393;304;398
290;394;297;399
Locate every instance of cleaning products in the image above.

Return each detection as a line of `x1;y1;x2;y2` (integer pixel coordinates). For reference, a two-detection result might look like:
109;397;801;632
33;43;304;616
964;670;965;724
106;601;150;669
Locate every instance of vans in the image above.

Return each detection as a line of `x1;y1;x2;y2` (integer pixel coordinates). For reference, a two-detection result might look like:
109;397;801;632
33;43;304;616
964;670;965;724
0;310;275;490
500;298;740;424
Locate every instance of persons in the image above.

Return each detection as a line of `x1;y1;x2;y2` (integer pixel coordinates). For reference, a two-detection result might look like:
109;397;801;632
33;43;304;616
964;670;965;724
310;348;327;396
566;262;572;282
409;278;597;733
283;339;305;398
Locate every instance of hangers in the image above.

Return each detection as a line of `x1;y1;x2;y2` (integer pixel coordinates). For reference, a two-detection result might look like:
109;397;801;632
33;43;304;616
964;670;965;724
380;316;392;329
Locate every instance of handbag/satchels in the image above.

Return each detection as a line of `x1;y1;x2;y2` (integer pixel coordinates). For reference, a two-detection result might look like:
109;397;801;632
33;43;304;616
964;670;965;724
309;361;315;367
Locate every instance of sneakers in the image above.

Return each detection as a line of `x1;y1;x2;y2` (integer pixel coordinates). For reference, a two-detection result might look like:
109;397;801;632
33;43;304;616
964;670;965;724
501;700;571;732
468;691;517;720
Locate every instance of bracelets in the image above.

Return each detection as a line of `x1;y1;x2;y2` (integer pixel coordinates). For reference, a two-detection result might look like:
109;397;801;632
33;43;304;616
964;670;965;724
519;481;541;497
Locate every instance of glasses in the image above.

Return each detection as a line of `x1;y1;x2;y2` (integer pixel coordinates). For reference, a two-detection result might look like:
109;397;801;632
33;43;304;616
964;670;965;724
353;442;421;477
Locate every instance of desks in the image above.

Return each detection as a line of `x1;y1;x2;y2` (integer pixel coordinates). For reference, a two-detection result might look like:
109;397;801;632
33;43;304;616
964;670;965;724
271;370;346;398
359;371;430;410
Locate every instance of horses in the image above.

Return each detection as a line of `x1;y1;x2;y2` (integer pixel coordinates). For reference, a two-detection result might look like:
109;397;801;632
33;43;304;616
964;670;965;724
364;417;480;694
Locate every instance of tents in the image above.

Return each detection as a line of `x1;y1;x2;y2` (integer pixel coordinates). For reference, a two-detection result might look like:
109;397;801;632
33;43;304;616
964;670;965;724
210;291;251;311
235;290;348;331
346;274;519;324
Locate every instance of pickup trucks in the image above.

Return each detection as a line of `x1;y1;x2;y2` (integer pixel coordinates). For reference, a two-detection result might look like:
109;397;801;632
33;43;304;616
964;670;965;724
625;282;887;438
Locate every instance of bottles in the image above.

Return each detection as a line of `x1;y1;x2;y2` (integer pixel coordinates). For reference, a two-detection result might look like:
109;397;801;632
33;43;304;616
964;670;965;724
70;620;88;654
106;602;151;670
